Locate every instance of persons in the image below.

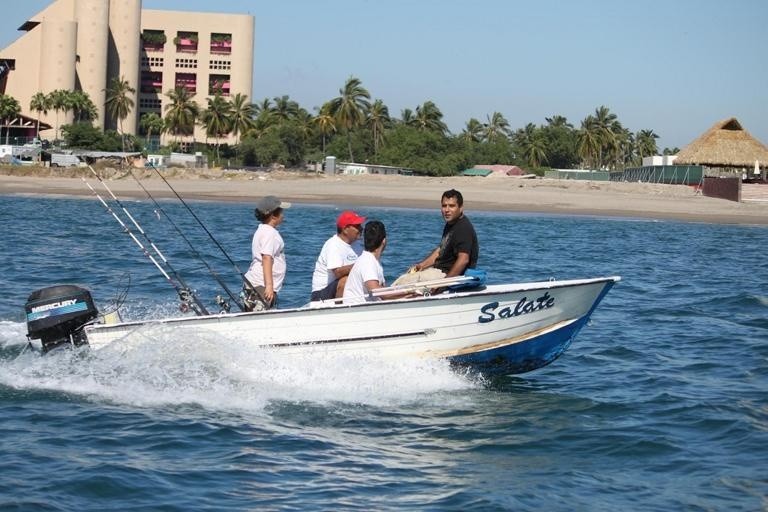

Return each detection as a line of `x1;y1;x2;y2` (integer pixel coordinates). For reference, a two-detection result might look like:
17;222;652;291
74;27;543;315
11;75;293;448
340;220;423;306
306;210;368;305
240;195;293;312
389;187;479;299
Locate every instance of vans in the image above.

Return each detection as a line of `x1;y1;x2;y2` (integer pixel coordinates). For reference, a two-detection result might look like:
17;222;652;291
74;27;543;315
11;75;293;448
51;154;80;168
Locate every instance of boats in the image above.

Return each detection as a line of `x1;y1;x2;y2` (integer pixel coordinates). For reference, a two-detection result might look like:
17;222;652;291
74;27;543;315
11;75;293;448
24;275;620;377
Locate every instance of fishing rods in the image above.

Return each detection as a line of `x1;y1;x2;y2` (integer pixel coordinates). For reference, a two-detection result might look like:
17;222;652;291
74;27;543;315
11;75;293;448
75;124;269;316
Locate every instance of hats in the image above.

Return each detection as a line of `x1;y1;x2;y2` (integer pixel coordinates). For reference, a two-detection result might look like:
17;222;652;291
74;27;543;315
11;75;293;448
256;195;291;216
336;210;368;230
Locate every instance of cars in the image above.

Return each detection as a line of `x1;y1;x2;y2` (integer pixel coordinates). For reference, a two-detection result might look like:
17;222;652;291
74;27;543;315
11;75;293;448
23;140;42;148
